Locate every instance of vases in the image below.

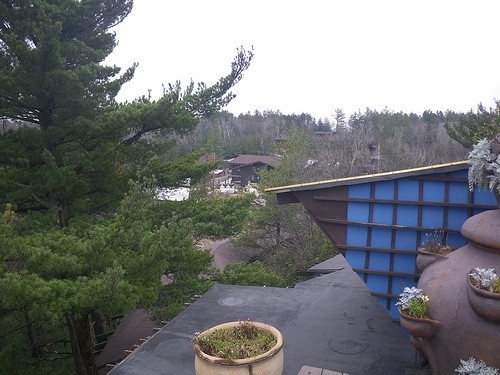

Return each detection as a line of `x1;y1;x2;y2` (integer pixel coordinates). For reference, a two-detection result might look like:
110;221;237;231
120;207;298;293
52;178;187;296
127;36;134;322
415;242;447;271
468;268;500;321
191;318;286;375
397;304;444;338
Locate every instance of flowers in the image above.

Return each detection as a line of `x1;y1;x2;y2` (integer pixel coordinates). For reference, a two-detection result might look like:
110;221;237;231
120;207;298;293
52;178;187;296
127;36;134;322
395;286;430;315
467;138;500;194
469;267;500;293
455;356;500;375
